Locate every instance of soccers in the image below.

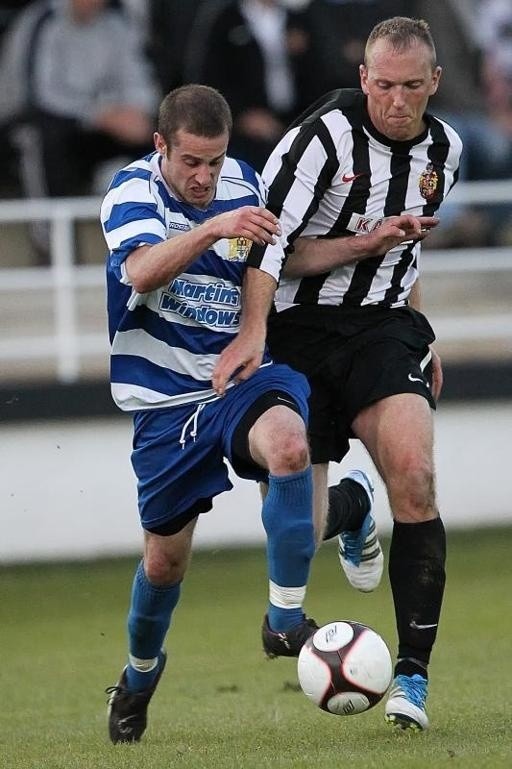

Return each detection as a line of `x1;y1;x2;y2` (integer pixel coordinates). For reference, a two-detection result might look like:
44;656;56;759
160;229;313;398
297;620;393;716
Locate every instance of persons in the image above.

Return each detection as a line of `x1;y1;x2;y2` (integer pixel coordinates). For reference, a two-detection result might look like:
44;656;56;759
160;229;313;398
208;14;465;734
97;84;443;746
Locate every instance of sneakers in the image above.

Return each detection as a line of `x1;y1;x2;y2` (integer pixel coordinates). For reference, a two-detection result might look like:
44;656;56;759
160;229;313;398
261;614;319;659
107;647;167;745
385;674;429;732
337;468;384;594
